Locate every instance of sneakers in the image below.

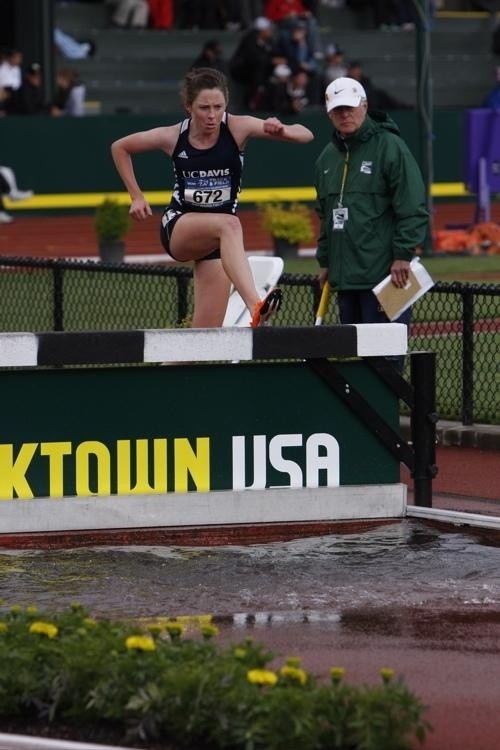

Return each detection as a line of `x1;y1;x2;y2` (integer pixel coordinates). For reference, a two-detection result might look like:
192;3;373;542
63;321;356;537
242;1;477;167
250;287;284;328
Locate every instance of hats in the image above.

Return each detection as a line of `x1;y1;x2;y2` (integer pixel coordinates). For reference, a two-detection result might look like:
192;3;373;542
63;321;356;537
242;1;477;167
324;76;367;113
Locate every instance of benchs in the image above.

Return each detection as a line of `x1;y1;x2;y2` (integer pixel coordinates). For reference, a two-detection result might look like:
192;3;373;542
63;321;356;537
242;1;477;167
19;0;500;114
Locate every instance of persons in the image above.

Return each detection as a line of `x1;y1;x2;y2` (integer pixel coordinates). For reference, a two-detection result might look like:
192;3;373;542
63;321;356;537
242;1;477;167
109;67;314;368
108;2;175;32
312;76;431;359
1;27;132;117
0;162;35;225
190;1;399;118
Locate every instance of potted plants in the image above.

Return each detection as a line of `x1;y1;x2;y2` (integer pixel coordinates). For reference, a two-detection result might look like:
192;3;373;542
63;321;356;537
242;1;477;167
259;199;314;260
95;196;131;264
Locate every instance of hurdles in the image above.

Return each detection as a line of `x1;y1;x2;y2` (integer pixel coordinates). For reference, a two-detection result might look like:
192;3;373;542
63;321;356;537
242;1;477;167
0;322;439;535
